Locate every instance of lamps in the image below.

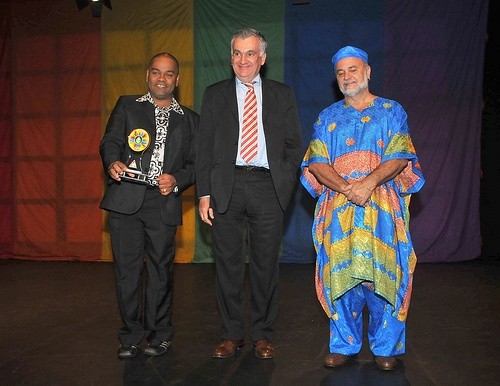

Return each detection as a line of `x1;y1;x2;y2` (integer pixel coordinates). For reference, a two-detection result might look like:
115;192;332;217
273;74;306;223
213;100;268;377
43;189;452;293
76;0;112;17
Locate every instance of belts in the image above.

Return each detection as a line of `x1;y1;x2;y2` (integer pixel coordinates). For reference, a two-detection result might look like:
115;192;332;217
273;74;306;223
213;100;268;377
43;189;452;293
236;166;256;173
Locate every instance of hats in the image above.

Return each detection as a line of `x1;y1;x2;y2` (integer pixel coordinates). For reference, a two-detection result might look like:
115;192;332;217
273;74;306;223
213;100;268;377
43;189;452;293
332;46;369;69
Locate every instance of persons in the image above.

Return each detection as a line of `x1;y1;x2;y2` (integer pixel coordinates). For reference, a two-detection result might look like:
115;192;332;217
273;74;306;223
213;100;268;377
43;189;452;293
300;46;427;372
99;52;200;359
196;28;303;360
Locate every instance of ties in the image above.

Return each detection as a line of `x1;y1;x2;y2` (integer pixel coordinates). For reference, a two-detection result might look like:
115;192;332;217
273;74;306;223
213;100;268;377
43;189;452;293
240;82;258;164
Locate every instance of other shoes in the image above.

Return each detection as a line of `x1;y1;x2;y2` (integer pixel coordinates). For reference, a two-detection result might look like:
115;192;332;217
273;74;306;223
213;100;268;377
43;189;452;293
117;343;142;358
143;338;172;355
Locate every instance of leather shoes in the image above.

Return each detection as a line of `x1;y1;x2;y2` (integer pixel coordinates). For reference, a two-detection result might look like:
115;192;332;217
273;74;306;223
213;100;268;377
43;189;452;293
252;340;273;358
375;356;397;371
324;354;358;368
212;338;244;358
